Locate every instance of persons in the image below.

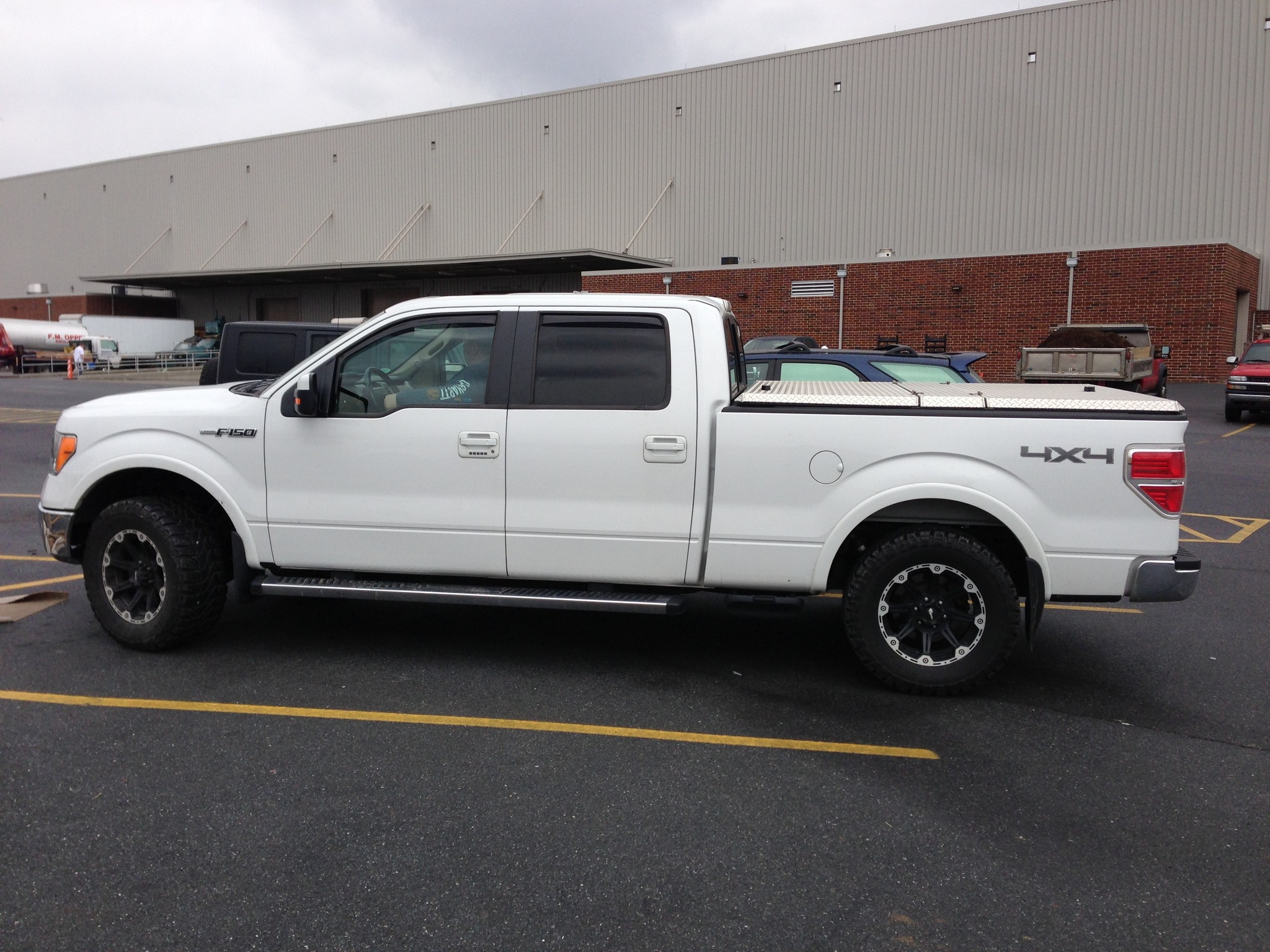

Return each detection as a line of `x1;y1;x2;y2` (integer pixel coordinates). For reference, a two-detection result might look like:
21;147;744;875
384;328;493;410
74;343;84;361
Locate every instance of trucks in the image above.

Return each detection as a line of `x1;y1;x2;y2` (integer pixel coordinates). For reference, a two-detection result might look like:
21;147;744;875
0;318;121;374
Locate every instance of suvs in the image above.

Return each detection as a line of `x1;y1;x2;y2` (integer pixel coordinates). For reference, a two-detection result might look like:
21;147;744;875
155;338;220;365
742;341;988;383
744;336;818;381
199;317;466;387
1225;340;1270;422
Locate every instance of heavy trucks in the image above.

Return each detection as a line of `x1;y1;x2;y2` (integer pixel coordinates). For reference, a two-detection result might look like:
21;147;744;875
58;314;195;367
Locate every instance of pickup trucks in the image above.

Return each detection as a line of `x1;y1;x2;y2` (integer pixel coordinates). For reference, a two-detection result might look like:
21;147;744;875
35;294;1201;691
1018;323;1170;396
